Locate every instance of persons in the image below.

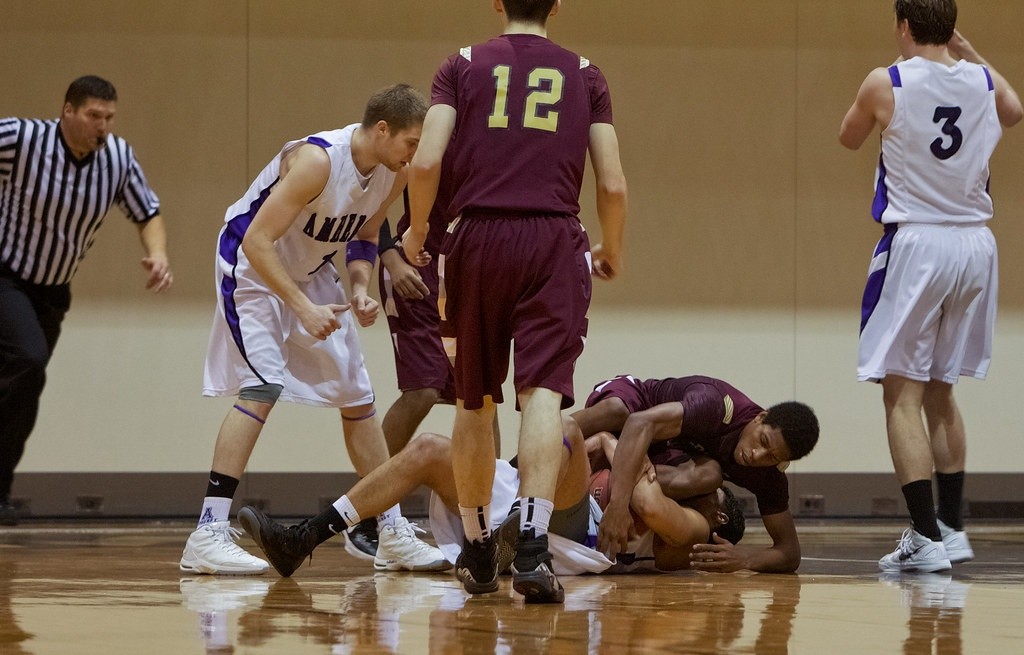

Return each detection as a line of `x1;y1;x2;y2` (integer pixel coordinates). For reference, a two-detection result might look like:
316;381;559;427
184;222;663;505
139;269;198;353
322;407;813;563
179;84;451;575
839;0;1024;571
1;76;174;515
341;127;501;558
404;1;628;603
511;375;821;572
237;432;746;575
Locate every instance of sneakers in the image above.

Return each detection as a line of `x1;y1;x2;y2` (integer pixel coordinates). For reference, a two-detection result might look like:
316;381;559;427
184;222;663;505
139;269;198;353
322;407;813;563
180;521;270;575
341;518;379;560
454;507;524;583
455;530;500;594
879;519;976;572
509;527;565;604
374;517;453;572
237;505;319;578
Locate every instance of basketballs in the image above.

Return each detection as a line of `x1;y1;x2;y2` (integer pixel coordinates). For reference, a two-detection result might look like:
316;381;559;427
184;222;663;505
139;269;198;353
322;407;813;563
588;467;646;541
